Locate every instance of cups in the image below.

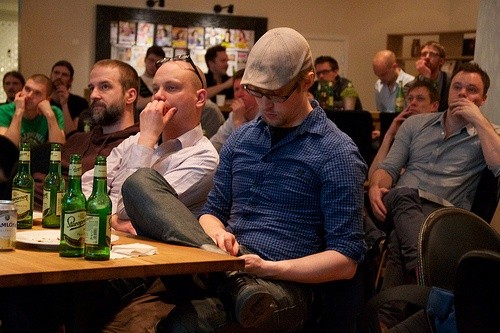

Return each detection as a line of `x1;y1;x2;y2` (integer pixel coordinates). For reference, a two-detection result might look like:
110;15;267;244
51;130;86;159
216;95;225;107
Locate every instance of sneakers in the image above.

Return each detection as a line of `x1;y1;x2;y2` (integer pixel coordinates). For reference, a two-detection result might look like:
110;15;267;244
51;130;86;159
226;272;273;330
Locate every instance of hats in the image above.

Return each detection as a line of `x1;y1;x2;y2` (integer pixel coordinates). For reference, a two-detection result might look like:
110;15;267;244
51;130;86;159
239;27;312;91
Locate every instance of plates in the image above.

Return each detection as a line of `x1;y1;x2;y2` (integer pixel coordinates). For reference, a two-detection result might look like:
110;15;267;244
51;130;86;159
15;230;119;247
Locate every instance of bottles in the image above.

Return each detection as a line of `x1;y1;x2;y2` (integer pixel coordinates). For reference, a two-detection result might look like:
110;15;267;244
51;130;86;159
325;82;335;111
396;81;405;112
59;154;87;256
84;155;113;261
11;143;35;229
41;144;66;228
342;82;356;110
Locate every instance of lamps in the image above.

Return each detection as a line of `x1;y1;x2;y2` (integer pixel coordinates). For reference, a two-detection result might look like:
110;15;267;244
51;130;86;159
146;0;165;8
213;4;234;13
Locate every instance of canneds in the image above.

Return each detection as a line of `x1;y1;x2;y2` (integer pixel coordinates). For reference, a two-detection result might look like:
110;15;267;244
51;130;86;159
0;200;17;251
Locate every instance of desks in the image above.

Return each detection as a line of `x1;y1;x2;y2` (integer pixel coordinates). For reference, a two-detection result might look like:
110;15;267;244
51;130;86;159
0;210;245;333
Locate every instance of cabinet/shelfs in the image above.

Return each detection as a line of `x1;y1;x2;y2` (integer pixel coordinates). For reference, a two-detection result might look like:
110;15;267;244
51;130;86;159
386;29;476;81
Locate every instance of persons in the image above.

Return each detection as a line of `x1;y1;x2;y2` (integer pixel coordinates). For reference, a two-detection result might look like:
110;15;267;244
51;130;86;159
121;27;368;333
308;56;363;111
373;49;415;113
81;54;220;333
364;63;500;293
203;44;262;154
403;41;450;114
142;45;166;97
0;59;141;210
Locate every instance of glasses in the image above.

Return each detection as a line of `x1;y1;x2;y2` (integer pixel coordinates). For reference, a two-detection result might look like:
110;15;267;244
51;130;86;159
316;69;332;76
421;51;440;58
244;82;300;104
155;55;204;89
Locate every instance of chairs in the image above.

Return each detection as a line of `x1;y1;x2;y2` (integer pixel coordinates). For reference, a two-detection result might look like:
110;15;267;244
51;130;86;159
373;170;500;288
418;206;500;309
